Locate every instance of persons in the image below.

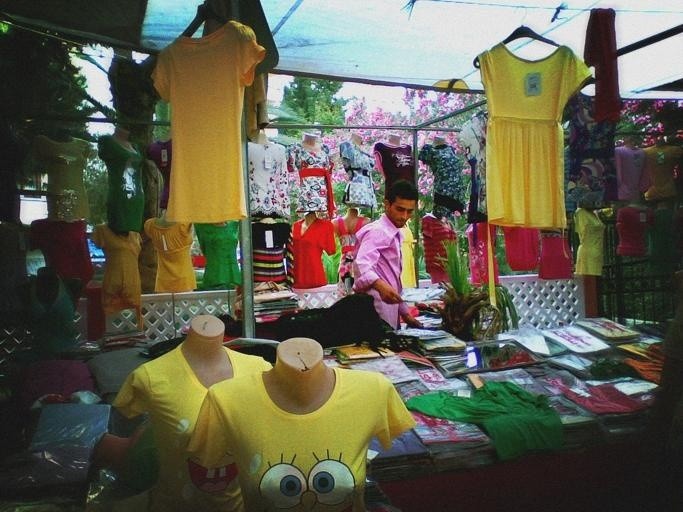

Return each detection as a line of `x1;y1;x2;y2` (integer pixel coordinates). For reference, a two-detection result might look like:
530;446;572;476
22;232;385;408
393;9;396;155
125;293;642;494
247;132;683;290
111;313;274;512
187;336;417;511
0;125;241;358
351;179;425;352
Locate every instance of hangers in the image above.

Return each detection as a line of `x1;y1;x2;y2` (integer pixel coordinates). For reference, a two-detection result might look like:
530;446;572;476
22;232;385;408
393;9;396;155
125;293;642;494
472;6;574;70
156;0;256;61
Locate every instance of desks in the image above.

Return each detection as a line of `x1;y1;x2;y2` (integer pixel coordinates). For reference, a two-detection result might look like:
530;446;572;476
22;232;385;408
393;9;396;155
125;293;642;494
0;322;662;512
